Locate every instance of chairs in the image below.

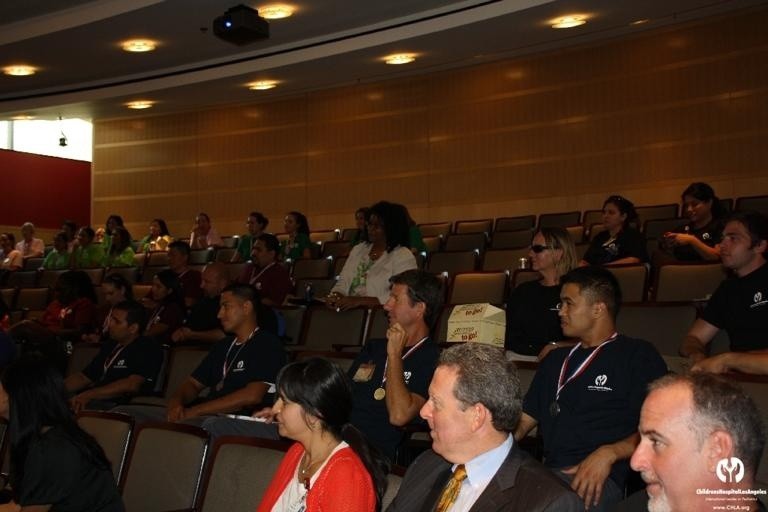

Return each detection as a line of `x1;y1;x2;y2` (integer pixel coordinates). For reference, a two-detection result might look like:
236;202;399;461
0;196;768;512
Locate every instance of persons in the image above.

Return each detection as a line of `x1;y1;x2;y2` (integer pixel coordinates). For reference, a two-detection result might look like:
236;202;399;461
0;182;768;512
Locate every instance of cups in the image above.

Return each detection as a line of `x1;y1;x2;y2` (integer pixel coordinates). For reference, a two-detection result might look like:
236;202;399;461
327;292;340;312
518;257;532;268
58;308;74;328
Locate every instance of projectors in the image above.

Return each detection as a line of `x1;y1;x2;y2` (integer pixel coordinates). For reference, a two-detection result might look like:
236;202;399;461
212;4;269;47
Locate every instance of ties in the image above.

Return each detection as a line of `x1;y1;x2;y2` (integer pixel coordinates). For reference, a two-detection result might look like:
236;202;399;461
435;465;467;511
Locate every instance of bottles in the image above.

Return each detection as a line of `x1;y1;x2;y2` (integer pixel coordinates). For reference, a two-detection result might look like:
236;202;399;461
303;280;314;301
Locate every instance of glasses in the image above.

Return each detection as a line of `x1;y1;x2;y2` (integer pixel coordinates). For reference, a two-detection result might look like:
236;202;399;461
532;244;558;254
